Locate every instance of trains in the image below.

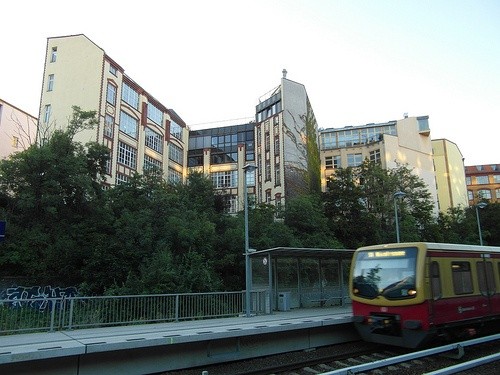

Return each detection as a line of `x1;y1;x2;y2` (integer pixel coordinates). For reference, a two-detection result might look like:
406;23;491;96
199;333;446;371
348;241;500;347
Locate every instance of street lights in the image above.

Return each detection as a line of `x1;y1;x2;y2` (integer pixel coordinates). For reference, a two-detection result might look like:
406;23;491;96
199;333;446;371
242;163;260;317
475;202;486;246
387;191;405;243
